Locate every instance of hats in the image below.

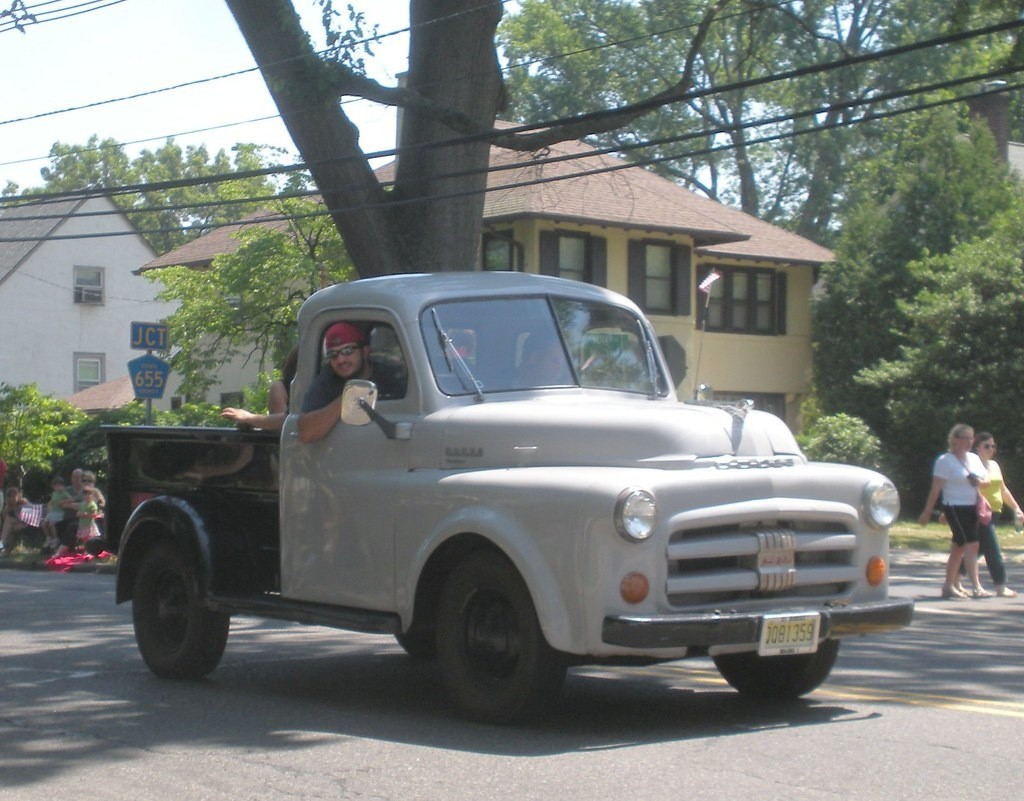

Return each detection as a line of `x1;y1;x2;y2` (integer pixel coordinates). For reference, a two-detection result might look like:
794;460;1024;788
325;323;364;346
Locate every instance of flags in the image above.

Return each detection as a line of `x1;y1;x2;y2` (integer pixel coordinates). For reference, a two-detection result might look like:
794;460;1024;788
18;502;42;528
698;272;722;292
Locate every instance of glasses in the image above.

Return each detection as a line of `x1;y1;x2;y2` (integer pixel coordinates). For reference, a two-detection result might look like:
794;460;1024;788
326;345;361;360
81;479;93;483
956;435;975;441
983;443;995;450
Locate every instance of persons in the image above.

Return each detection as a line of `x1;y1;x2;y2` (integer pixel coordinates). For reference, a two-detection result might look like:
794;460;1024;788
218;343;298;430
298;322;412;441
938;431;1024;596
505;330;574;391
919;423;996;599
0;458;107;563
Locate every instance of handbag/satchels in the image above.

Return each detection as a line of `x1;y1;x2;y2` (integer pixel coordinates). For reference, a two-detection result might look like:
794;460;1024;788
976;494;992;526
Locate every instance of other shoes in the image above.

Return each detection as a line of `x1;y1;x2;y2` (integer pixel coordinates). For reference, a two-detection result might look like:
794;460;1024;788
974;588;995;599
956;584;972;596
43;536;60;548
942;586;967;600
995;586;1017;598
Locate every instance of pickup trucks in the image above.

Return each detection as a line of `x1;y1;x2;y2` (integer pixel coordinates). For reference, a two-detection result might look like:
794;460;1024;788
100;271;914;727
